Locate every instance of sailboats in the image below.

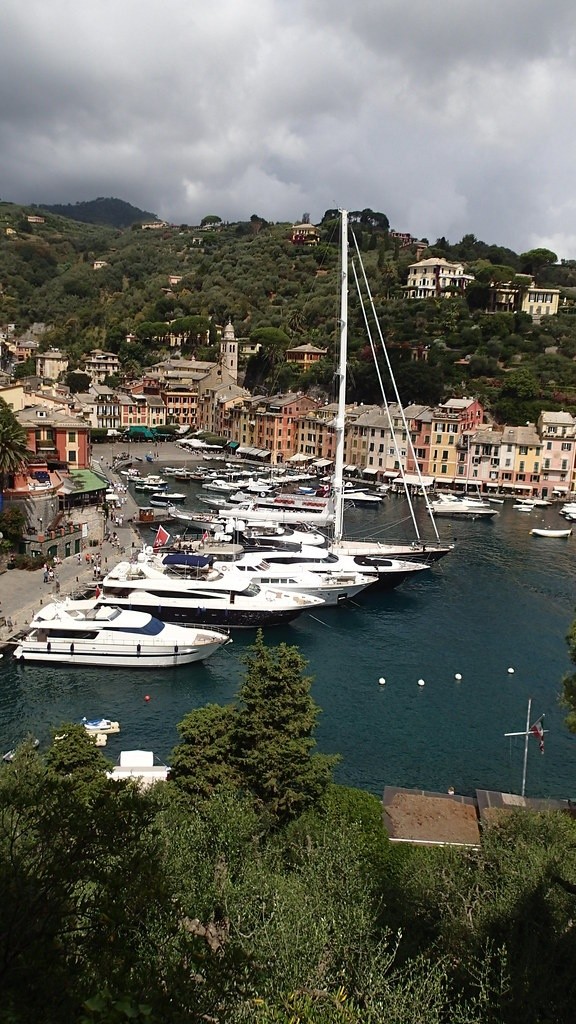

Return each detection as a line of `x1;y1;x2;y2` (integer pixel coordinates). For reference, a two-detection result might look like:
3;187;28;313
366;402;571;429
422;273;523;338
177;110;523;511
171;208;455;565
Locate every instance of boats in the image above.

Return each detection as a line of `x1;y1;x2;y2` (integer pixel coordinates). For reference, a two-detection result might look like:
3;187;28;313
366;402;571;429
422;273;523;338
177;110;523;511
81;717;121;736
12;465;576;670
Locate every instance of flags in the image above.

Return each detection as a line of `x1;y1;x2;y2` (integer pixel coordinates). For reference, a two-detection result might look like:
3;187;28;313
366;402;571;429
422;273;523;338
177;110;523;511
532;720;544;754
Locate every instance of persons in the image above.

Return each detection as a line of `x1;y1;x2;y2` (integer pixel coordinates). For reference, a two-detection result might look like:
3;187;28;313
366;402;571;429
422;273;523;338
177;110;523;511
99;539;102;549
111;512;124;527
77;553;101;577
109;531;120;548
44;563;54;582
101;455;104;461
7;617;13;632
121;496;127;504
114;481;127;493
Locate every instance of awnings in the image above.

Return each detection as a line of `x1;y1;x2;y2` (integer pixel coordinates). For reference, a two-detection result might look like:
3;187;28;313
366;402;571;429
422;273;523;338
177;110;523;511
392;475;435;486
554;486;568;491
435;478;452;483
455;479;532;489
343;465;356;472
228;442;238;447
309;455;334;474
383;471;400;477
362;468;378;474
236;446;271;458
